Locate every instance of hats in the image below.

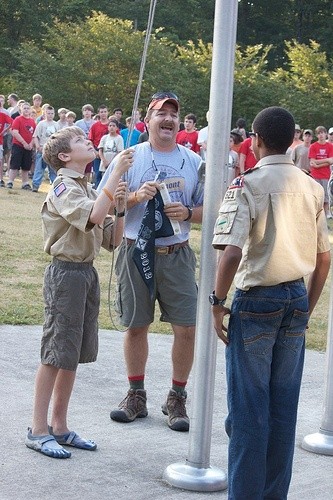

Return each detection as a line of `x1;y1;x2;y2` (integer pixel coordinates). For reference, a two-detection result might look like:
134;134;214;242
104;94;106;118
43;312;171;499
328;127;333;134
147;95;179;113
231;129;243;136
304;129;313;134
295;124;301;131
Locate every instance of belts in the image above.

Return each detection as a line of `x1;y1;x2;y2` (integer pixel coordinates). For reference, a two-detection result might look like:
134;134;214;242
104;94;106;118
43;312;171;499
124;238;188;254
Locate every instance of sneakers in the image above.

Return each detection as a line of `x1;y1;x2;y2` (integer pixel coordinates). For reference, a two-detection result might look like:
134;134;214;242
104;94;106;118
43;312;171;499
160;389;190;431
110;388;149;422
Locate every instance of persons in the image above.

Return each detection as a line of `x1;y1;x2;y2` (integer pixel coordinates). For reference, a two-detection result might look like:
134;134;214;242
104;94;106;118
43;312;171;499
209;107;331;500
96;98;205;432
287;124;333;218
0;94;148;192
228;119;258;184
25;127;135;459
176;112;208;161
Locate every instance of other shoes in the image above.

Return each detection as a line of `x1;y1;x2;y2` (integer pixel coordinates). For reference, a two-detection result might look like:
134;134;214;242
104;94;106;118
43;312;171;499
7;183;13;188
0;180;5;187
22;184;30;189
32;186;38;191
48;425;97;451
25;427;71;459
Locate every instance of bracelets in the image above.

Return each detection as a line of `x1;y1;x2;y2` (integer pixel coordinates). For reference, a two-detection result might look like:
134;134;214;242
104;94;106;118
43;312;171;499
183;206;192;221
133;192;140;204
113;207;126;217
103;188;114;201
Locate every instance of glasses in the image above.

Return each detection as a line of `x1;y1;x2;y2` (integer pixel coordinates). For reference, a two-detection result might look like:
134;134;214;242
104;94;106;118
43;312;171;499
250;132;264;140
147;92;180;107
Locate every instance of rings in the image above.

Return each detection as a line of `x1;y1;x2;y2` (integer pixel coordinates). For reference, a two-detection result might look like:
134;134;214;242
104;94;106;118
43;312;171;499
175;213;177;216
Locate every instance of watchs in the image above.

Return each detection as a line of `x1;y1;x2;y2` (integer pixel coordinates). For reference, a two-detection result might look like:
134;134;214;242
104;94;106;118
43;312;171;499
209;290;227;305
